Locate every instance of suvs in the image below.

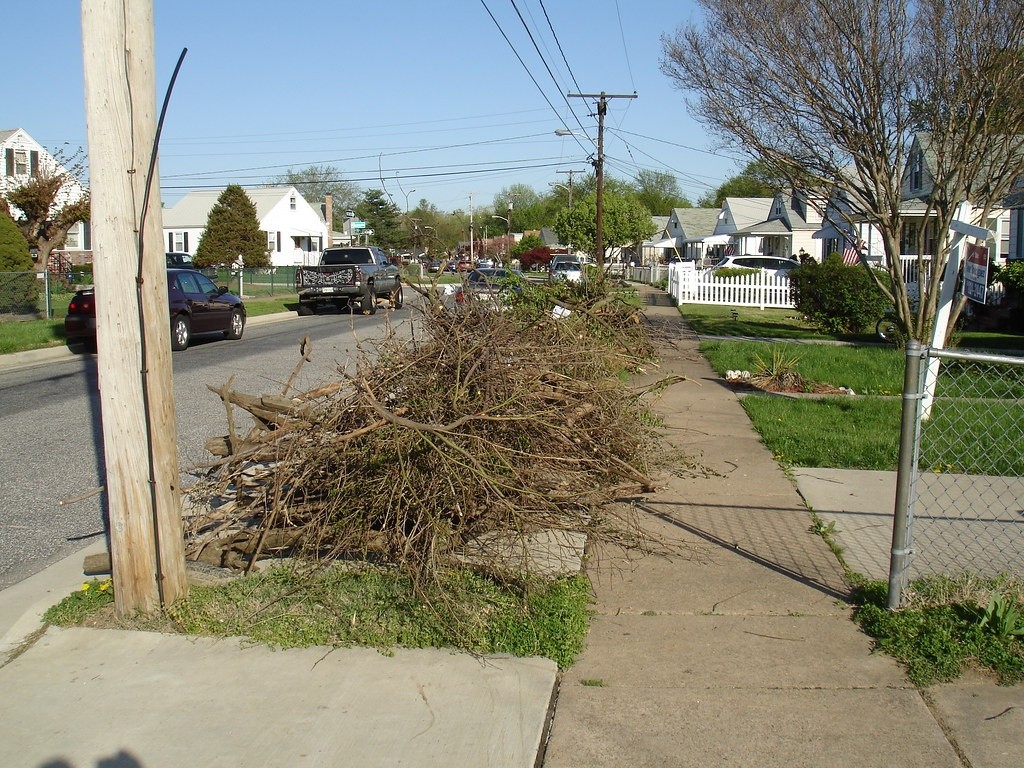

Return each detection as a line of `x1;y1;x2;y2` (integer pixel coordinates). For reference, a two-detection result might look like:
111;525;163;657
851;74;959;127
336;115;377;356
550;253;582;286
713;253;801;276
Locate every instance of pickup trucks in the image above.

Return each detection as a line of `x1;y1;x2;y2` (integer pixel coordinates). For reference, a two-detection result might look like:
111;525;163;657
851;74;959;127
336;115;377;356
294;246;403;317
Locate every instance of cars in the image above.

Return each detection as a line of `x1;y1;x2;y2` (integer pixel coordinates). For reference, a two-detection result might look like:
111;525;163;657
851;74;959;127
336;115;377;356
166;251;197;270
427;258;492;273
455;267;523;311
64;268;247;353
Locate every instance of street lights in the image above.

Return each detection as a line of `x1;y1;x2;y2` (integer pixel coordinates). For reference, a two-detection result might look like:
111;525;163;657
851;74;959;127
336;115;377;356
554;128;604;277
424;225;438;264
490;215;511;270
